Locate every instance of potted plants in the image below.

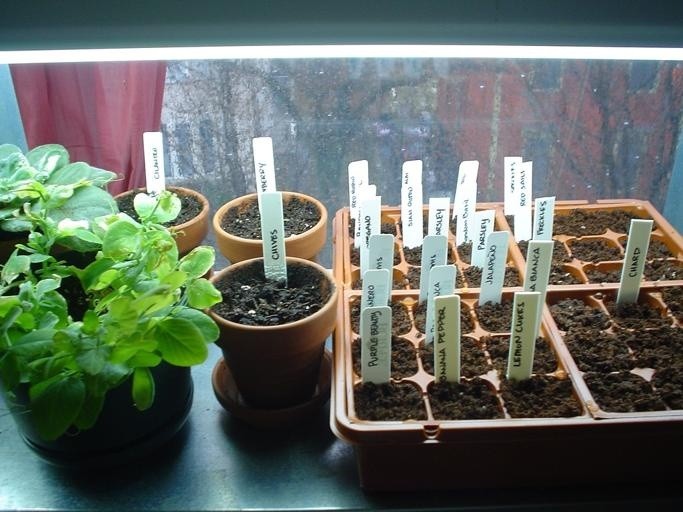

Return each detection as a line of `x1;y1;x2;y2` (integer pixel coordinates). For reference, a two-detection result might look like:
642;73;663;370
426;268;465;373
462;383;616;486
0;142;225;485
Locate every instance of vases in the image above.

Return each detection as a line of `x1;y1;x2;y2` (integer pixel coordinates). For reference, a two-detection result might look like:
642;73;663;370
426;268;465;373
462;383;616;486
108;186;210;257
211;190;329;262
201;253;340;430
